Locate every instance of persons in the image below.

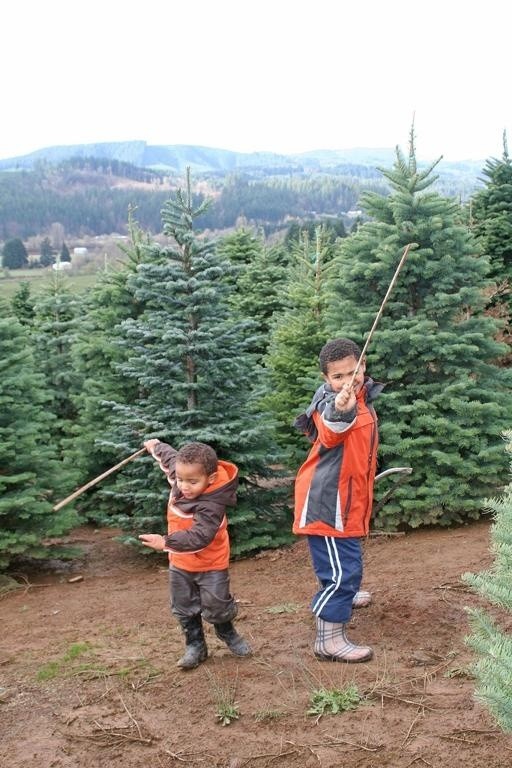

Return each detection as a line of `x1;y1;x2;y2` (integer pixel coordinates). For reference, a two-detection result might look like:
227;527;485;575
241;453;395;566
138;438;253;669
289;339;387;662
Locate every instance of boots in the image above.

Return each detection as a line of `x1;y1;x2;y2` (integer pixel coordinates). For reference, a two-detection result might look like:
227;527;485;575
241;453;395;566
214;620;253;658
351;590;373;610
175;625;209;669
312;614;375;664
